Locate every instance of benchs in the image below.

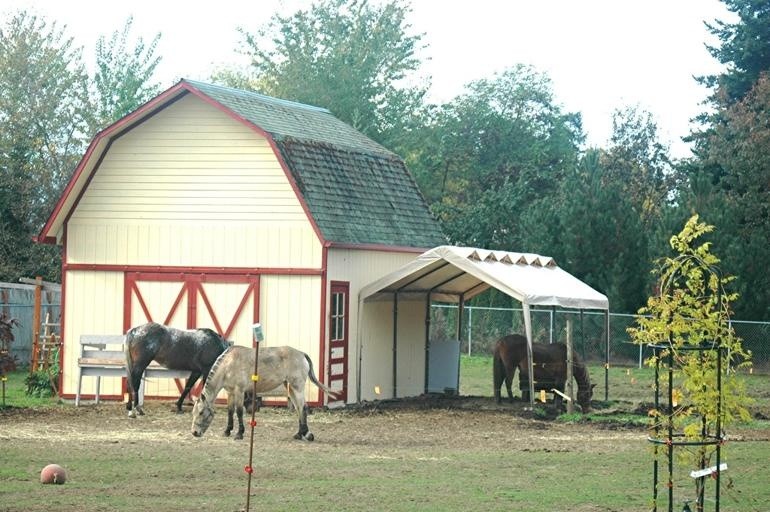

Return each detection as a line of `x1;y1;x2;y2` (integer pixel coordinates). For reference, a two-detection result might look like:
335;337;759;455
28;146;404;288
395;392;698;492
74;335;204;409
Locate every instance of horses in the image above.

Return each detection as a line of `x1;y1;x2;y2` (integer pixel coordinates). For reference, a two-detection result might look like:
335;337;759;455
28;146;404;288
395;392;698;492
122;322;263;418
489;335;598;415
190;345;342;441
